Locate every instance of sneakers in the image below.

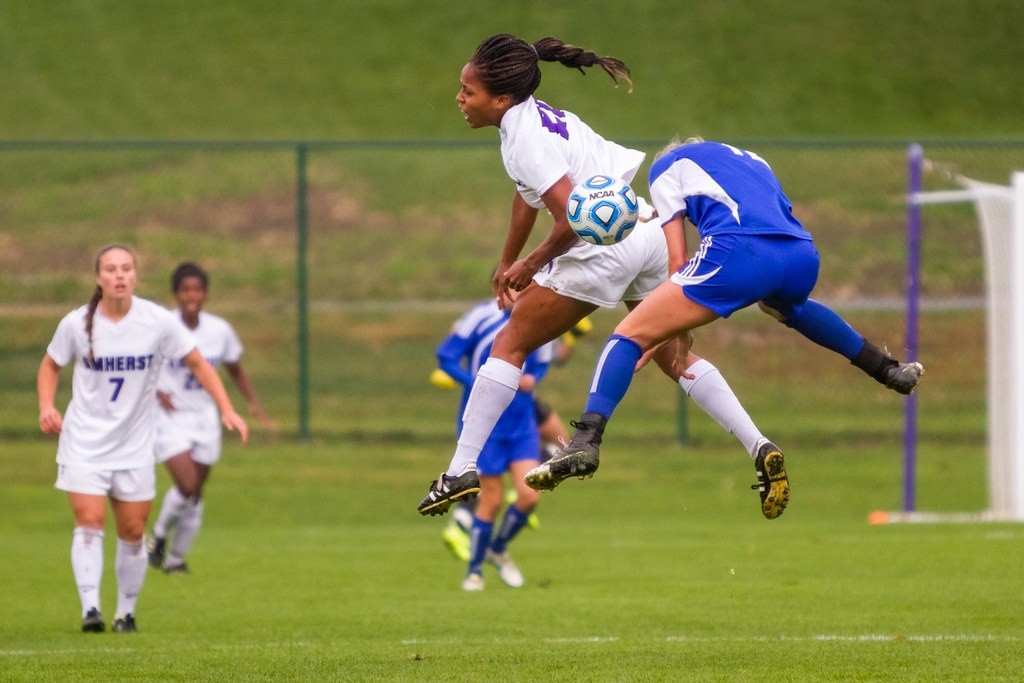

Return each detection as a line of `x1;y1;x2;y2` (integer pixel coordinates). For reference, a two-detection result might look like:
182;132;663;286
417;460;480;517
751;437;790;519
524;436;599;493
884;342;924;394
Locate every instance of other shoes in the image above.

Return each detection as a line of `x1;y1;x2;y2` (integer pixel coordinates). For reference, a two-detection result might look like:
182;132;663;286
505;489;539;530
442;519;470;562
111;613;136;632
484;542;523;588
162;552;187;575
462;562;484;592
81;607;105;632
147;524;166;569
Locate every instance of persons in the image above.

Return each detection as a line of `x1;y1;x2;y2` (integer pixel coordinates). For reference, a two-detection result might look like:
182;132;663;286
417;33;790;520
146;262;274;578
436;262;593;593
524;134;924;492
37;246;249;633
